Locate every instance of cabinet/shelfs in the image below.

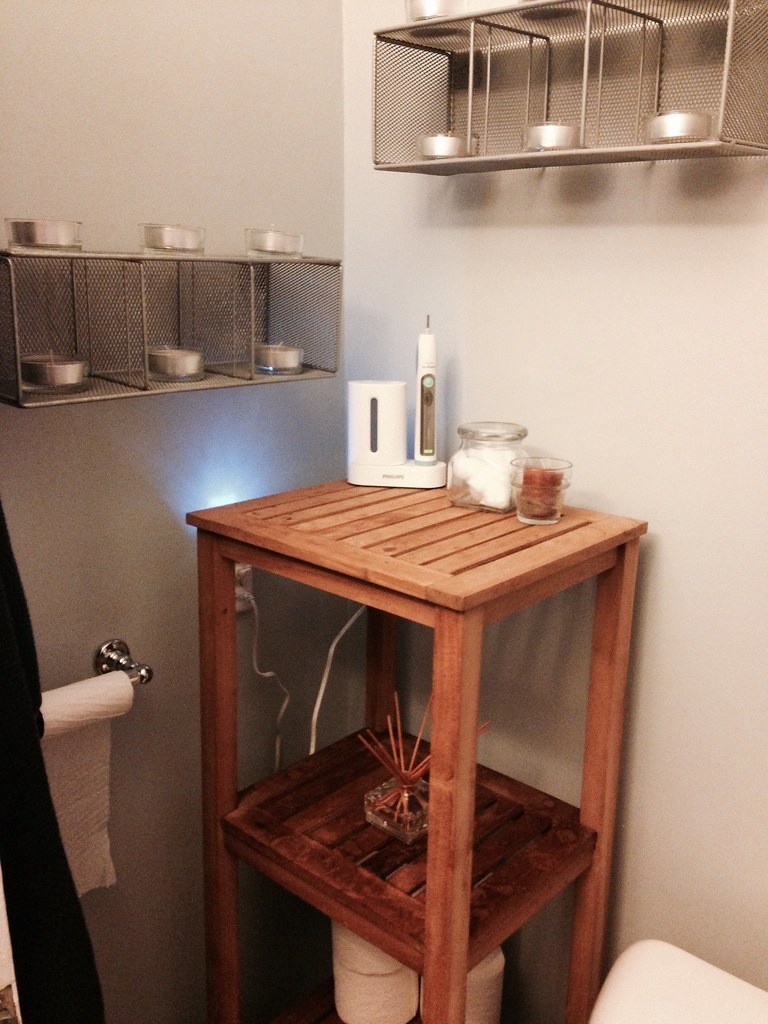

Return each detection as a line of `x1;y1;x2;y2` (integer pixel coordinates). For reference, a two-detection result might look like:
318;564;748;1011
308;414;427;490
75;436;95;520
186;480;647;1024
373;0;768;175
1;245;341;408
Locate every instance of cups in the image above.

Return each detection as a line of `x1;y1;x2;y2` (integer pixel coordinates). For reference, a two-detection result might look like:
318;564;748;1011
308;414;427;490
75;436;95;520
510;457;573;525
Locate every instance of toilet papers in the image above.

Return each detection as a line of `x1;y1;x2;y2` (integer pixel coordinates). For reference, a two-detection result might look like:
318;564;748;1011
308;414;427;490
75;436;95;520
38;668;135;741
330;916;506;1024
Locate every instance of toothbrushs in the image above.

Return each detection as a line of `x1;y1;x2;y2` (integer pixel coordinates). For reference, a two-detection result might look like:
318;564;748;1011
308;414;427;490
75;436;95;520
412;314;440;467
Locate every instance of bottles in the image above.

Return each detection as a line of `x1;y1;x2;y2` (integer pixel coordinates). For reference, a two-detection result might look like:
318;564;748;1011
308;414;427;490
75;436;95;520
447;422;529;514
